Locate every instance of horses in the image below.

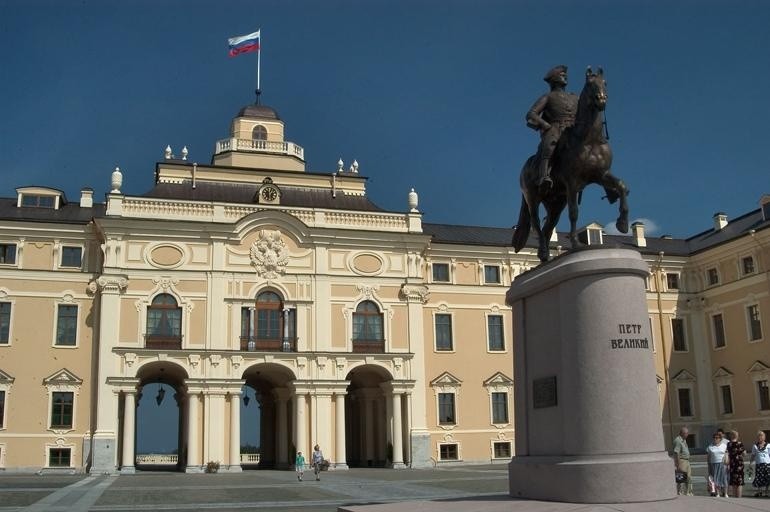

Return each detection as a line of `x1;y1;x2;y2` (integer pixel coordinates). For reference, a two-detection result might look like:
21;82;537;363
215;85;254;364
511;65;629;262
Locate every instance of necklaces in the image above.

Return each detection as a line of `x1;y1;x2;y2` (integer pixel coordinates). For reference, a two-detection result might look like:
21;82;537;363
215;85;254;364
312;444;324;481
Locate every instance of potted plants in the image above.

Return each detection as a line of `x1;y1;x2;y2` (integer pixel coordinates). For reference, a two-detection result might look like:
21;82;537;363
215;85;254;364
207;460;222;474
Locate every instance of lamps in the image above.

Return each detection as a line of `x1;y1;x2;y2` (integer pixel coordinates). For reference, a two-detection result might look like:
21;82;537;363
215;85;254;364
155;378;162;407
243;375;250;409
158;367;166;402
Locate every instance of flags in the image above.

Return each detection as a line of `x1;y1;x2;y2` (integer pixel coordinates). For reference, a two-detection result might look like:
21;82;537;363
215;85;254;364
227;32;258;56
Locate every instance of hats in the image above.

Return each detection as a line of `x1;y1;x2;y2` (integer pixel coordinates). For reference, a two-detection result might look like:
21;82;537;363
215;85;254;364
713;431;724;438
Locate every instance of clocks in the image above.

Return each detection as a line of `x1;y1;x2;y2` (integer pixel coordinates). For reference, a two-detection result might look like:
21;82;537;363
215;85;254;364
262;186;278;203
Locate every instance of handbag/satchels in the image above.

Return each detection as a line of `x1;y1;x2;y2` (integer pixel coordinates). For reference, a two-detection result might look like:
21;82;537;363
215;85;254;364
708;480;716;493
676;471;687;483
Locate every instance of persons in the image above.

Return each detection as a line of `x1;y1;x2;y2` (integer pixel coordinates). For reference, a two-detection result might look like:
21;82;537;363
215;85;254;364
525;64;582;194
673;425;770;498
295;451;308;481
